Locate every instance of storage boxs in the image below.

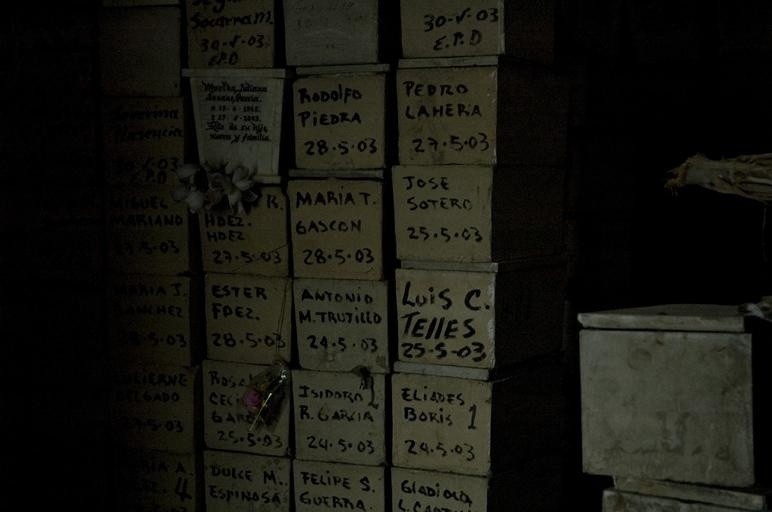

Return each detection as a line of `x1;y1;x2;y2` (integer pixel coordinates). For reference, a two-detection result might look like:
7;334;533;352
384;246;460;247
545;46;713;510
293;371;386;463
203;451;290;510
183;5;277;70
199;360;291;459
400;2;554;58
86;0;201;508
180;70;285;181
394;64;567;164
387;467;558;510
195;184;289;276
286;68;390;169
292;461;386;509
599;476;763;511
294;279;388;372
203;283;293;367
391;167;565;271
291;169;383;275
576;306;757;487
393;269;565;374
282;1;379;61
388;375;558;478
